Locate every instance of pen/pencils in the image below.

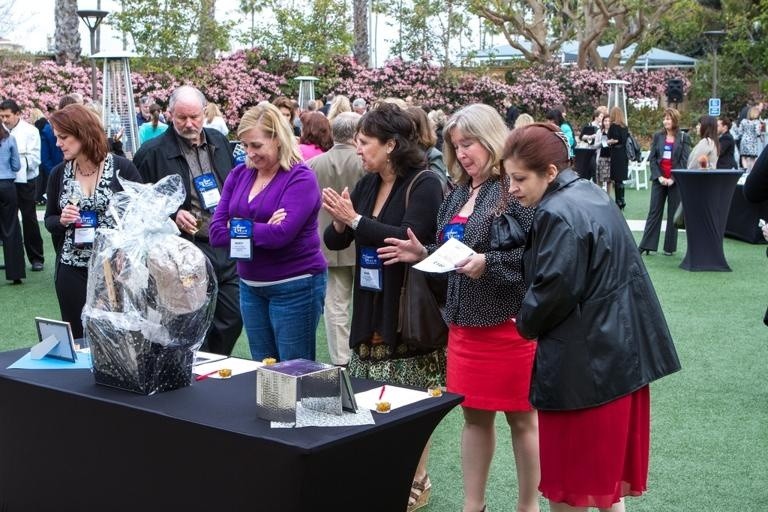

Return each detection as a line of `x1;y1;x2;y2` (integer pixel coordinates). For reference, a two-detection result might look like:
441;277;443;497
379;384;386;400
195;370;219;382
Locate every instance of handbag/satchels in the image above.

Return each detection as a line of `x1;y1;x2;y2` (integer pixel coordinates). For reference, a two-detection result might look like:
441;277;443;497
400;168;447;357
488;211;525;250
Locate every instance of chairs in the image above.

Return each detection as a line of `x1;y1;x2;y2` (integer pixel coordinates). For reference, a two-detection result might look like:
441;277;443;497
628;150;650;190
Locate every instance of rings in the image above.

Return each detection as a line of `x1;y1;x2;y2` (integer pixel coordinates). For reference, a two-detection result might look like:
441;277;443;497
180;224;185;229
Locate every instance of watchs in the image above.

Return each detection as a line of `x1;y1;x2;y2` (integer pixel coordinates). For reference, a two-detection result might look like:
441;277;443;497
351;215;363;230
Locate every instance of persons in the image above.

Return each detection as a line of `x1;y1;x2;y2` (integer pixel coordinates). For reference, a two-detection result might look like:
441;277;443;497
546;109;574;166
574;110;604;181
686;114;721;170
402;107;448;198
135;85;245;358
736;99;763;173
203;102;229;137
376;103;541;511
138;104;168;146
35;104;56;205
208;103;328;363
500;121;680;512
2;100;45;272
42;96;77;178
637;108;690;256
716;115;739;170
43;102;143;335
0;121;28;286
606;107;628;211
600;113;613;195
743;143;768;329
136;96;167;127
85;102;125;156
295;110;334;161
352;98;366;115
272;97;294;134
307;112;365;371
321;99;446;512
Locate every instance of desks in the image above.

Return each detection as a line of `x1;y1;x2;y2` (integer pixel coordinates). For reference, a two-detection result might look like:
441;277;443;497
669;170;744;272
1;338;464;511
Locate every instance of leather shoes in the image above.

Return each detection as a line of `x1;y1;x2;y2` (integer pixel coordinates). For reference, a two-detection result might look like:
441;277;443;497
24;258;48;272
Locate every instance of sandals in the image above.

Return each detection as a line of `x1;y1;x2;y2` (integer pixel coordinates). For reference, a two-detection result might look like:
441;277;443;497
408;472;433;510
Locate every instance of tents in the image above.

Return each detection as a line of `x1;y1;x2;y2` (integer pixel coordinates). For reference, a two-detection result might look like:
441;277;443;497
457;40;534;58
565;41;699;78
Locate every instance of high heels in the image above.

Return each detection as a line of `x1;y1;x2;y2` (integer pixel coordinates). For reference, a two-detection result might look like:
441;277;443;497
635;243;652;257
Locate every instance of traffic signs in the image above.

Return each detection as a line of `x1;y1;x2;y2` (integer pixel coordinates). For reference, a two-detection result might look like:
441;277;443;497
709;98;721;116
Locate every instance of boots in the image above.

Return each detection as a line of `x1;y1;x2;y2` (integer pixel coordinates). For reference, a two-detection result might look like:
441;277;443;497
613;177;629;212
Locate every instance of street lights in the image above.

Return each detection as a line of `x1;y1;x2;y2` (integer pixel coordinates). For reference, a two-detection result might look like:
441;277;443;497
78;11;109;103
76;8;110;96
704;27;726;99
704;31;725;115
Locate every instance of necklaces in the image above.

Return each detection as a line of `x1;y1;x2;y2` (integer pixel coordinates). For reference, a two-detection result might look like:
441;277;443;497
75;161;98;177
469;180;486;196
262;171;276;190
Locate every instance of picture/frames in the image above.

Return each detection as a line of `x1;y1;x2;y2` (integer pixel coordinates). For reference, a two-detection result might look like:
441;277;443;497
30;317;75;363
